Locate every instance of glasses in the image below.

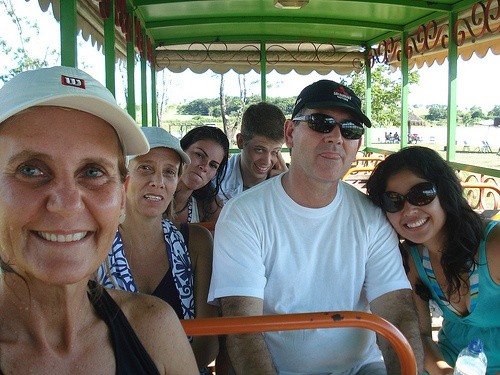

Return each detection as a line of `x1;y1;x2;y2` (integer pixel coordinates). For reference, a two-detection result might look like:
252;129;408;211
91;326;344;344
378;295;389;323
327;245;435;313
292;112;365;140
378;181;437;213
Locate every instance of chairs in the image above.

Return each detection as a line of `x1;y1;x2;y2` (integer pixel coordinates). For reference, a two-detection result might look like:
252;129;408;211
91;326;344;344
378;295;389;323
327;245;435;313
462;141;492;153
430;136;434;144
343;155;385;184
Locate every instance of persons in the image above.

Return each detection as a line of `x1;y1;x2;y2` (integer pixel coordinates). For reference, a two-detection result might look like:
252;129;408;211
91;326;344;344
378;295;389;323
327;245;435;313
0;65;202;375
385;132;418;143
94;101;290;375
365;146;500;375
205;79;428;375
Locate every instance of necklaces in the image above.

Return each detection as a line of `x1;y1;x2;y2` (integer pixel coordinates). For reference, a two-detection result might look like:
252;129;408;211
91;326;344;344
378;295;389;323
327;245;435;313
173;200;189;213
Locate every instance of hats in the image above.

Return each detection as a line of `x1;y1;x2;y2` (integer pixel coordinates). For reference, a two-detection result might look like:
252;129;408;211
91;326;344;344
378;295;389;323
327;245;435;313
126;126;191;166
0;66;150;156
291;78;372;128
